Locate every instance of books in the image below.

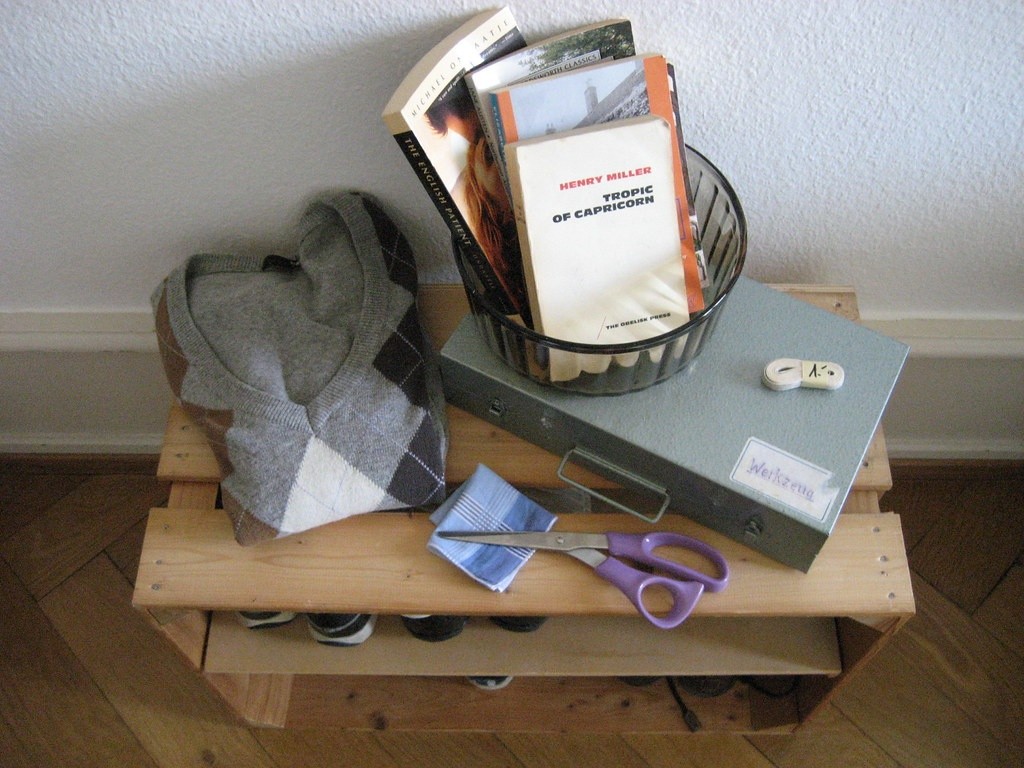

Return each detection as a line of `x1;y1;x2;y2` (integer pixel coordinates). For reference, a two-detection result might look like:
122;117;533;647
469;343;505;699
382;5;710;386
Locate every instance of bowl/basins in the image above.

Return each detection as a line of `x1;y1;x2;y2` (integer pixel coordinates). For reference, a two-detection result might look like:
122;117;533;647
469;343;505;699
450;142;747;397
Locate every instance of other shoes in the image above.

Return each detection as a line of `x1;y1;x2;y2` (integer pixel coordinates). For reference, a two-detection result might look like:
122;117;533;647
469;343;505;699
239;611;291;628
742;675;796;699
490;616;547;630
621;676;656;688
399;614;467;640
677;676;730;697
306;613;376;646
471;676;512;692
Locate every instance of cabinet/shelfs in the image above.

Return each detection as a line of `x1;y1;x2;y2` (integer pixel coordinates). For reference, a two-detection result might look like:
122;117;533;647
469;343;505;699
131;289;918;738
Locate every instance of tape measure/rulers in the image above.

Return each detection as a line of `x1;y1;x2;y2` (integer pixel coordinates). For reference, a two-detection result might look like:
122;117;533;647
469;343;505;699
762;357;846;392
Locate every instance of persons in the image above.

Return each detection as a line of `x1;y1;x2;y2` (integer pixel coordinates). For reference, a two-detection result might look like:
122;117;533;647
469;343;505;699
426;69;481;143
462;132;531;307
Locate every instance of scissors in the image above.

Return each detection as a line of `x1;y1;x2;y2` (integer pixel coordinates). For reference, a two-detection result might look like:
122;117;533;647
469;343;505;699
437;524;732;630
